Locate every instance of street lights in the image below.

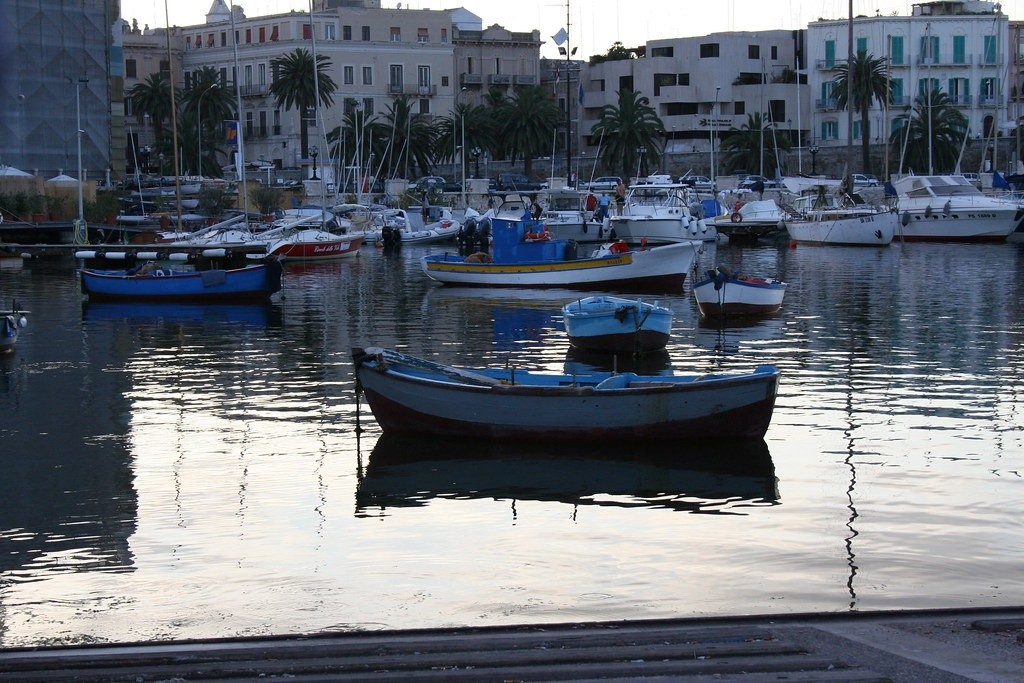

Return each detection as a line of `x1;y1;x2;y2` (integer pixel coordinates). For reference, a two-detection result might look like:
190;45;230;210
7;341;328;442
715;85;720;179
307;144;320;181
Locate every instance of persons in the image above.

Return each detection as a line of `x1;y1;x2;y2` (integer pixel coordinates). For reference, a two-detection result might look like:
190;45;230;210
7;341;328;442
615;179;626;216
421;191;430;225
571;170;576;186
587;191;611;223
370;174;374;186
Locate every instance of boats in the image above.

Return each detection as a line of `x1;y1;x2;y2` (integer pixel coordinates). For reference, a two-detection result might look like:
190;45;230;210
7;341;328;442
561;296;674;356
328;178;789;251
352;427;784;520
75;241;283;301
0;298;32;357
694;266;789;318
349;343;780;452
420;212;704;298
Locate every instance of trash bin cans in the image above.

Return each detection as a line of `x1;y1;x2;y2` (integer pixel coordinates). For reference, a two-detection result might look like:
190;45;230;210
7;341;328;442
682;180;696;188
327;183;334;194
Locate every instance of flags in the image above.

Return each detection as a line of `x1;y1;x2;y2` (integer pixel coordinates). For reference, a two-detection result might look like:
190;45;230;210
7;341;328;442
556;64;560;86
579;82;584;105
552;83;556;99
225;122;237;144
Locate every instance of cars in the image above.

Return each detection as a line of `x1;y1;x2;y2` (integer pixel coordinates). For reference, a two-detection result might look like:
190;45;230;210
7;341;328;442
410;177;462;193
467;161;984;195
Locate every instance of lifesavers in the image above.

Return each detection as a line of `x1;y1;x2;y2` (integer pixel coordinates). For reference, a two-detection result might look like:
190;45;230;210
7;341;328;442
526;230;551;242
731;213;743;223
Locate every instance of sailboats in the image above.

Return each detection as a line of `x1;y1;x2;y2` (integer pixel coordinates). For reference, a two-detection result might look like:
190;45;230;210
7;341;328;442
148;0;365;264
877;31;1019;245
779;0;901;248
